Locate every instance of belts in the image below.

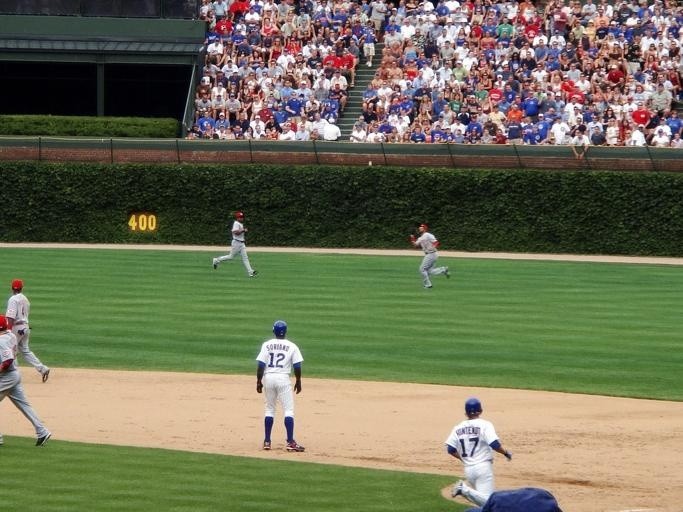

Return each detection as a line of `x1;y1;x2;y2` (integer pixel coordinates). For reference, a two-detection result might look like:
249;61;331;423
234;238;245;243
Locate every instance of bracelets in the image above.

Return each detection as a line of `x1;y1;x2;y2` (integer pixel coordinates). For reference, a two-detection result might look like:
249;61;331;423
257;382;262;386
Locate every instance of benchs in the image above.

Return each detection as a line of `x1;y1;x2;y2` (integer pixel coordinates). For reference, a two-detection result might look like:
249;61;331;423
196;0;682;156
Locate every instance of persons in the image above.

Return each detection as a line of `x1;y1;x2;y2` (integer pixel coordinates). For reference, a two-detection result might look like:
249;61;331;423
255;320;306;452
409;223;450;288
211;211;258;277
444;397;513;505
0;315;51;447
3;279;49;383
183;0;682;161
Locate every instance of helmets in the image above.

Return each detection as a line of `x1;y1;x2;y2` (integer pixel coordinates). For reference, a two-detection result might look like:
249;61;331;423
273;321;286;335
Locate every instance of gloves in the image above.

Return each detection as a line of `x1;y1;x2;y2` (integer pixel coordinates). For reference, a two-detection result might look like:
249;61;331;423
18;328;25;335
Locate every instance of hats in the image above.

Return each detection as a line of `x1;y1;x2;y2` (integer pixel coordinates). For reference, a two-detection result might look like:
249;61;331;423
236;213;243;219
197;3;682;147
465;399;480;415
0;316;7;329
420;225;427;231
13;279;23;289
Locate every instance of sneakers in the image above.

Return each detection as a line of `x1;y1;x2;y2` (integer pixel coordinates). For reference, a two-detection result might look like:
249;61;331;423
214;260;217;270
452;482;462;497
286;441;304;452
424;285;433;289
36;433;51;446
446;267;450;279
251;272;258;277
264;440;271;449
43;370;49;383
